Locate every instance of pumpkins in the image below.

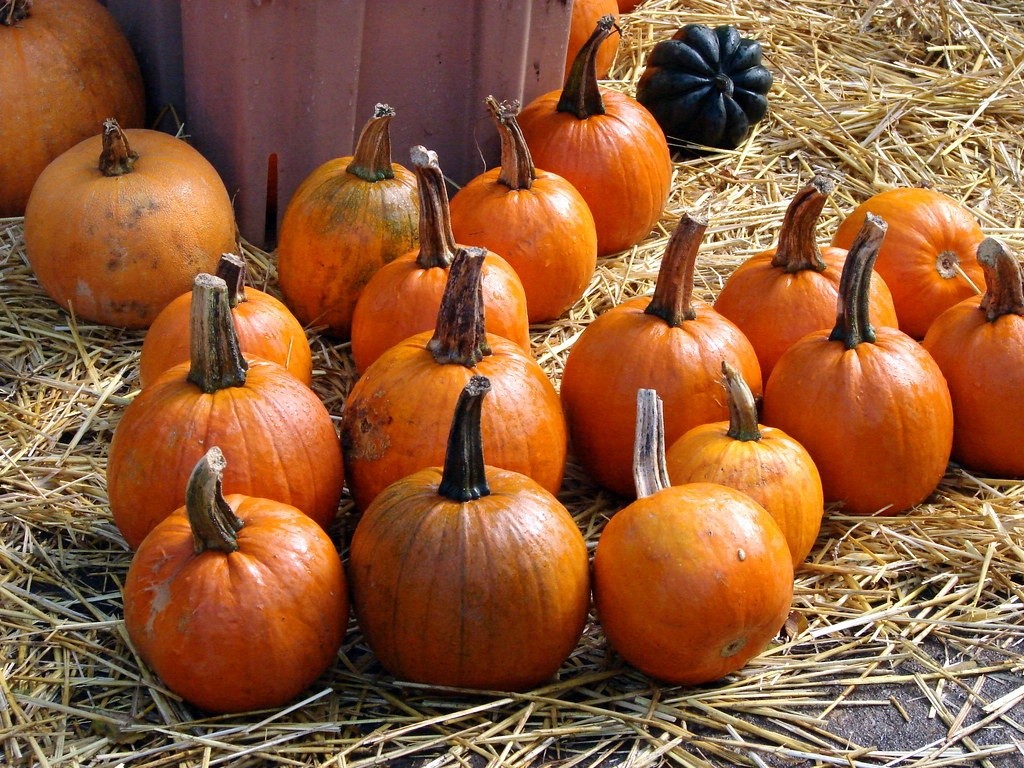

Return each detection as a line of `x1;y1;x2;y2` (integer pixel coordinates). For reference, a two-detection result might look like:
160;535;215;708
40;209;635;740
0;0;1024;711
